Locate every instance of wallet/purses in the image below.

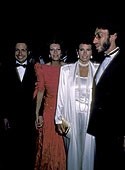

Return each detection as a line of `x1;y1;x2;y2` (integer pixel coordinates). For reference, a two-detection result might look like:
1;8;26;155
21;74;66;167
61;120;70;135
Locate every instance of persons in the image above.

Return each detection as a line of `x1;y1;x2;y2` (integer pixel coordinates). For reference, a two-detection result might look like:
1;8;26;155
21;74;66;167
86;20;125;170
54;36;101;170
0;39;36;170
33;37;70;170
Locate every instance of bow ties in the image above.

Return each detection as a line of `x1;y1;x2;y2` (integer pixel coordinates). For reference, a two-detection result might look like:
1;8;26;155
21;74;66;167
16;63;27;68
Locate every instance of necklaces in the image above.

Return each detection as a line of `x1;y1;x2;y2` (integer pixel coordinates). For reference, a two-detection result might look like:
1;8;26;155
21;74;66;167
76;59;90;67
